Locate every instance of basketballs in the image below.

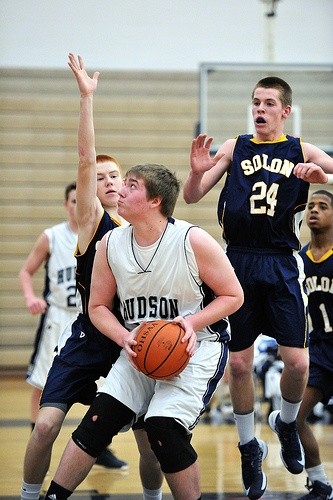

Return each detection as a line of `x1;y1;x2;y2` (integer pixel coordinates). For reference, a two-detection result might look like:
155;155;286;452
131;320;192;381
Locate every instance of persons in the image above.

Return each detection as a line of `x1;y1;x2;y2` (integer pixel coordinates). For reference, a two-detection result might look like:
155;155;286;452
20;181;129;474
201;189;333;500
184;77;333;500
20;53;165;500
42;163;245;500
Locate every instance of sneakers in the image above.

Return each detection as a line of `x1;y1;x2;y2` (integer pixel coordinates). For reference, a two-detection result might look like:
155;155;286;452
269;410;305;475
296;477;333;500
238;437;268;500
95;447;128;472
